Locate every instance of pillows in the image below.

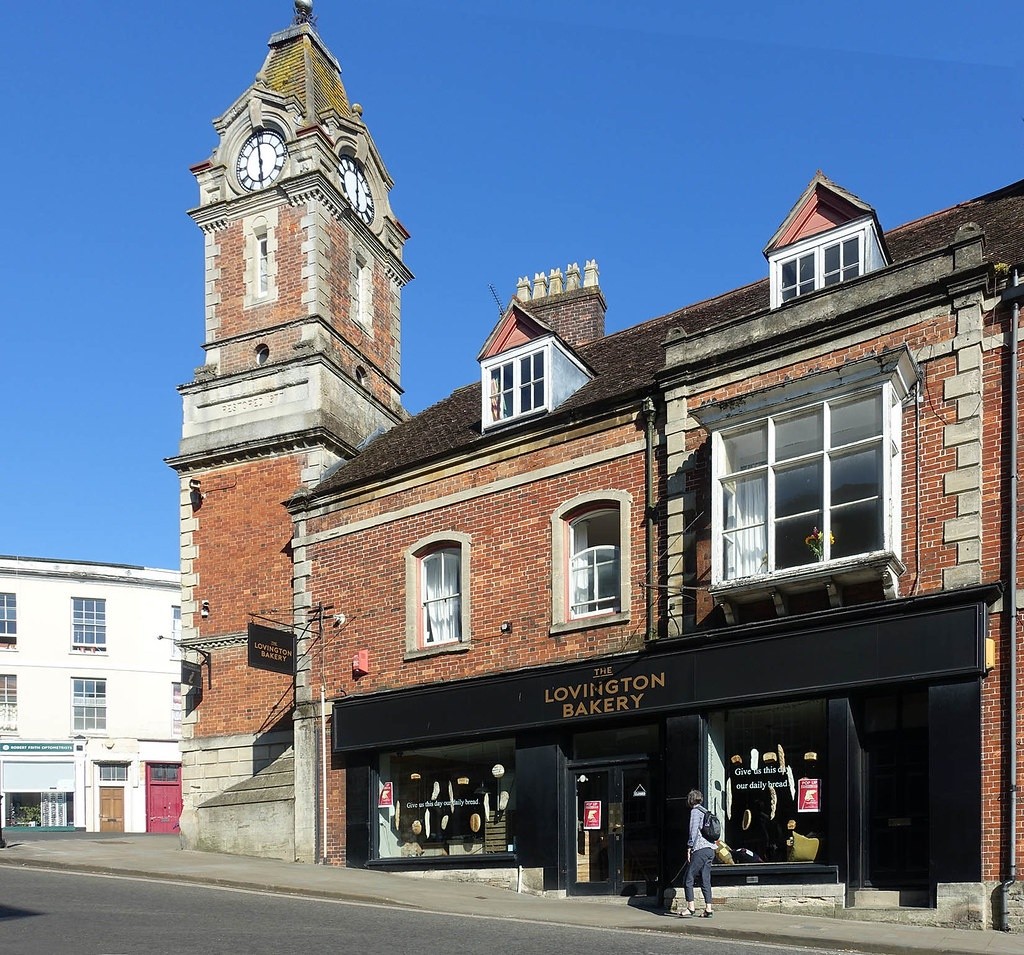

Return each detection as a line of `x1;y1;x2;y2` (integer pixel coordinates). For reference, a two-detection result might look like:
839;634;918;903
787;830;820;863
713;839;735;864
729;848;764;864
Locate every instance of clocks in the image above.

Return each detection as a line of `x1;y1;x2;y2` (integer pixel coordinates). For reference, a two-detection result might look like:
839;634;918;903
339;154;375;227
236;128;288;193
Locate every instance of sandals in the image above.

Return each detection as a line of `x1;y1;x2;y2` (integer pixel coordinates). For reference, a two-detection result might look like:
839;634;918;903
678;908;695;917
698;910;713;918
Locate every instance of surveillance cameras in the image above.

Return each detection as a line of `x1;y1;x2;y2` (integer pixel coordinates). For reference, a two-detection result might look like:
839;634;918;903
202;600;209;606
189;479;201;489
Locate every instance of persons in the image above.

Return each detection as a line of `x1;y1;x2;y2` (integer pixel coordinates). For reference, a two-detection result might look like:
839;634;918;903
678;790;717;919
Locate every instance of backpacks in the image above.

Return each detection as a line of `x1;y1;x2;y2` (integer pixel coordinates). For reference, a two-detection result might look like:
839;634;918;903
695;806;721;842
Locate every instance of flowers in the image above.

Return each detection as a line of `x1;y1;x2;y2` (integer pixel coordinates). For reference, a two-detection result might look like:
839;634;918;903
804;529;836;557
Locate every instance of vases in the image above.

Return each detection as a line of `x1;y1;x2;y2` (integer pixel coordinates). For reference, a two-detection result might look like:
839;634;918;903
818;555;824;562
